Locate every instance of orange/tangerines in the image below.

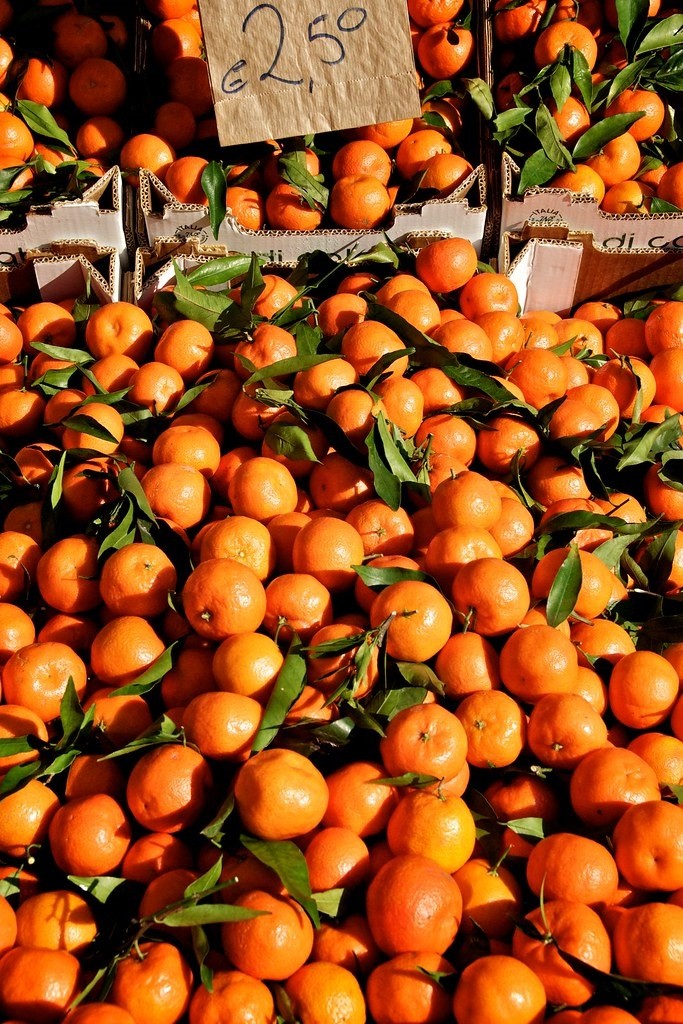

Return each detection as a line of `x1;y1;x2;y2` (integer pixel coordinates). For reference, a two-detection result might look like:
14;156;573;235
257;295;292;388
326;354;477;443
0;1;683;1024
